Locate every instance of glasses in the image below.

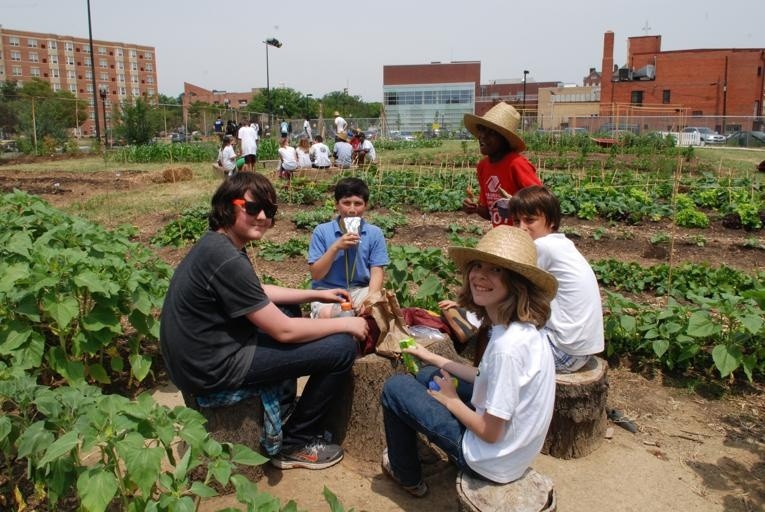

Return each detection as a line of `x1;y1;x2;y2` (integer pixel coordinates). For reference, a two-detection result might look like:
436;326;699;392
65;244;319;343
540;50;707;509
231;198;278;218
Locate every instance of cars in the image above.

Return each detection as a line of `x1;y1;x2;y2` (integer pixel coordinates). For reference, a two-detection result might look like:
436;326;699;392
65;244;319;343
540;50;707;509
352;121;608;141
138;121;210;145
681;126;764;145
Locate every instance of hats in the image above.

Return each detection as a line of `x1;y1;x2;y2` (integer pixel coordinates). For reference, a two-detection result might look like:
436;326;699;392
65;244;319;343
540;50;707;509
334;131;351;142
464;101;526;153
447;224;559;302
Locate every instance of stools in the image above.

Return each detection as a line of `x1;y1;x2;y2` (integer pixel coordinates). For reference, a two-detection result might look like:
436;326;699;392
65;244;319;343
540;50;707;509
456;468;557;512
183;387;267;496
540;356;608;460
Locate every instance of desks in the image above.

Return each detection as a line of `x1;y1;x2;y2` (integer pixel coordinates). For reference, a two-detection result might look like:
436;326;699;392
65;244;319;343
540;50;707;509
309;326;466;463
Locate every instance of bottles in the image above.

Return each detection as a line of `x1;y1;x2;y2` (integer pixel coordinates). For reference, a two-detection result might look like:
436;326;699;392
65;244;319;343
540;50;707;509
338;302;355;318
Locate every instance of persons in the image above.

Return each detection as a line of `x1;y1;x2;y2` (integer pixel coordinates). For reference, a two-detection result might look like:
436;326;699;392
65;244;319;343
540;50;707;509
308;178;391;320
382;225;558;498
438;186;605;372
160;171;370;470
463;102;543;227
213;111;376;177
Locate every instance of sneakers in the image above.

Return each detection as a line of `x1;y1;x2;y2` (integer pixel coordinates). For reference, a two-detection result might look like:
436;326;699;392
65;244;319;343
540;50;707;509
270;436;343;470
382;447;427;499
442;306;478;343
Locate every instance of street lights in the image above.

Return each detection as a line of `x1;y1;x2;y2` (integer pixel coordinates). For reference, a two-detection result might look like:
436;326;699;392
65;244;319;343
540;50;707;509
262;38;283;126
520;68;534;126
